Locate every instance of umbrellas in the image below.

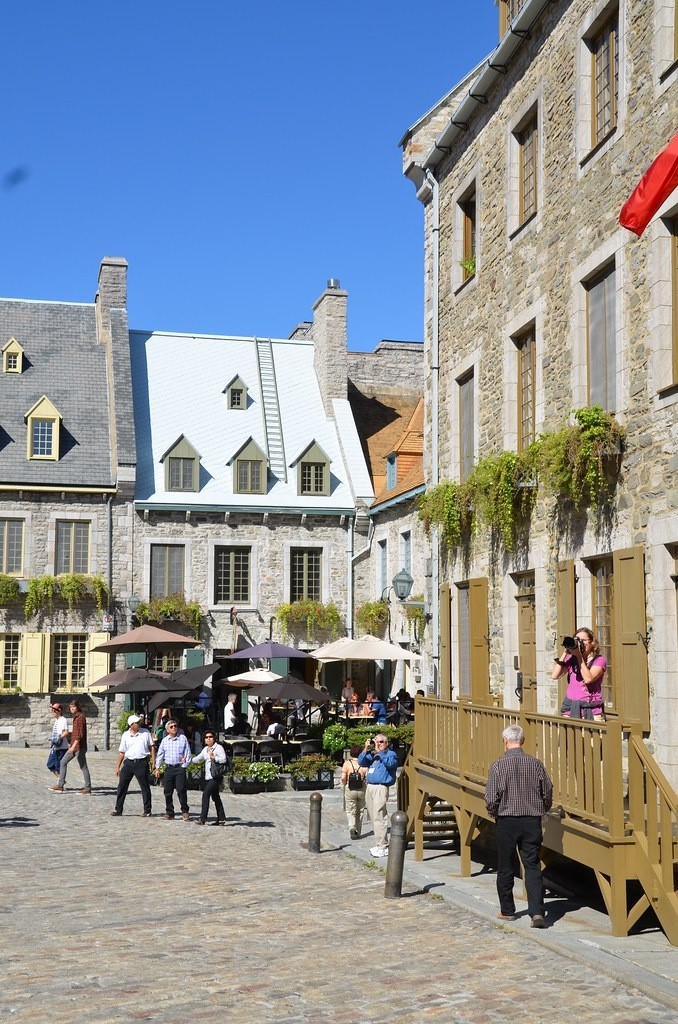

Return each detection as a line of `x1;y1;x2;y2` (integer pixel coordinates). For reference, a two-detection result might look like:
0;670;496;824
86;625;420;736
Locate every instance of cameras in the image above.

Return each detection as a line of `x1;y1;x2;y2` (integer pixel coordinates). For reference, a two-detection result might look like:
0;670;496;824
563;637;585;654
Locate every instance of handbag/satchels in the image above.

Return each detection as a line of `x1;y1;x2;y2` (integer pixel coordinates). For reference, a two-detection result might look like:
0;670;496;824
210;744;233;778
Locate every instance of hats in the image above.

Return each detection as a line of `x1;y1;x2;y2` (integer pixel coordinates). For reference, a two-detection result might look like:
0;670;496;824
49;703;63;711
127;714;140;725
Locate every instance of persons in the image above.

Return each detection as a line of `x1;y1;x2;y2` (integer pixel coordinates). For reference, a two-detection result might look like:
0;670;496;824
224;693;311;737
158;715;202;756
47;704;69;785
195;688;210;728
358;735;398;857
320;677;386;728
156;720;191;821
110;715;155;818
390;688;425;725
551;628;607;777
183;730;227;826
341;744;368;840
48;700;91;796
484;725;553;928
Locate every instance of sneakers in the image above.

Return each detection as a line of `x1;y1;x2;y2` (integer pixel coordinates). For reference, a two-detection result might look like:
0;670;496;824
369;847;389;857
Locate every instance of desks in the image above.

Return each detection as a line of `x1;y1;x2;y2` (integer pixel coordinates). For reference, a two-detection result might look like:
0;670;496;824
222;739;287;753
271;706;294;715
281;738;323;751
338;715;375;726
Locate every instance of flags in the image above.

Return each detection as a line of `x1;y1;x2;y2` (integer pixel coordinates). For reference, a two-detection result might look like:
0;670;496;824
618;133;678;236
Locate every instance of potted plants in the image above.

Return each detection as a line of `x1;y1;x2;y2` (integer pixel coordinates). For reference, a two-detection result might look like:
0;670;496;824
0;686;23;704
183;709;206;736
146;751;337;794
135;590;205;640
416;404;626;563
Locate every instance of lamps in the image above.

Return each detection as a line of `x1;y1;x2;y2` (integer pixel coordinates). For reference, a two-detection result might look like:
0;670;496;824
128;592;140;614
392;568;433;620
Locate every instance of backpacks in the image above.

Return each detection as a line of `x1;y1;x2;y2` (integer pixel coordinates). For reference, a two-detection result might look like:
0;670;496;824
347;759;366;791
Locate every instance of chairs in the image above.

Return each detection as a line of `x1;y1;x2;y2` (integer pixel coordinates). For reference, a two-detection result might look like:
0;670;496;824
219;724;323;773
248;700;263;728
316;701;401;726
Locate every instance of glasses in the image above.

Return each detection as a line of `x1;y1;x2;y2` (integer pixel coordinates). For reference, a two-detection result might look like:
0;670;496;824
169;725;177;728
378;740;387;743
204;735;213;739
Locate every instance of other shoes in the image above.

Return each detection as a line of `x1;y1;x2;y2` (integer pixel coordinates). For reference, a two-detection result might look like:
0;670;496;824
183;814;189;821
530;915;545;928
141;812;152;817
75;789;91;796
349;827;358;839
195;819;205;825
110;810;122;816
48;785;63;794
496;912;515;921
212;821;225;825
163;815;174;820
